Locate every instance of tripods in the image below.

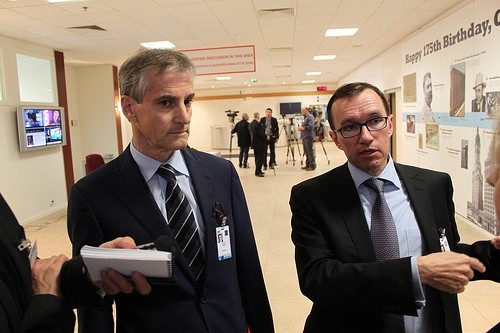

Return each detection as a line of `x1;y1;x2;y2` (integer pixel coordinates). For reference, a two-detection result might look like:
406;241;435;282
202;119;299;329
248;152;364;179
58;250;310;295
287;119;304;166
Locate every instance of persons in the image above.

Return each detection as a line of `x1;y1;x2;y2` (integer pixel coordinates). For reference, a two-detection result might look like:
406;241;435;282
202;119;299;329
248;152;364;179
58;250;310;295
472;74;488;112
260;108;280;170
231;113;251;168
310;108;325;141
249;112;265;177
0;194;151;333
487;108;500;248
289;83;500;333
422;73;435;123
297;106;317;171
66;49;275;333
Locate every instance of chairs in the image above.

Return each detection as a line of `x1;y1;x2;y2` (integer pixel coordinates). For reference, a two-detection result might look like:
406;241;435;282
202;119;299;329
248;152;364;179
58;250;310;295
85;154;105;175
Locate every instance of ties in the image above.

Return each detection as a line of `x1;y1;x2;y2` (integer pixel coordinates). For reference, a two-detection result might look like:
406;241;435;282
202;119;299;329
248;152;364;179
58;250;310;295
362;178;400;261
155;164;205;296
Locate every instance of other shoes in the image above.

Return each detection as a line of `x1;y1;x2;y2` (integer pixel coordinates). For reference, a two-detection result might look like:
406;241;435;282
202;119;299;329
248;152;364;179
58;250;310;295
301;166;315;171
269;166;274;169
256;172;265;177
263;167;267;170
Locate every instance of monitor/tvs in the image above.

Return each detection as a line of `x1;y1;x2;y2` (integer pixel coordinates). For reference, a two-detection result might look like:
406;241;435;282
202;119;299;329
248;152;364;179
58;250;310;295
279;102;302;117
17;105;67;152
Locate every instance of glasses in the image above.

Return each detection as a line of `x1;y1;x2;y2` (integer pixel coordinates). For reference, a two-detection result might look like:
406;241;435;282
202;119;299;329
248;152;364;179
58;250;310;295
333;116;388;139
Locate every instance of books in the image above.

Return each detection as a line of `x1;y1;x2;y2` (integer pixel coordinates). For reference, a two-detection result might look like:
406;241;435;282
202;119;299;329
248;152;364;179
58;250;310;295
80;245;173;281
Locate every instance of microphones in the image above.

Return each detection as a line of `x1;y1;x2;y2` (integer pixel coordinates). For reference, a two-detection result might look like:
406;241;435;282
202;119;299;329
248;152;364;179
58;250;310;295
136;234;173;251
